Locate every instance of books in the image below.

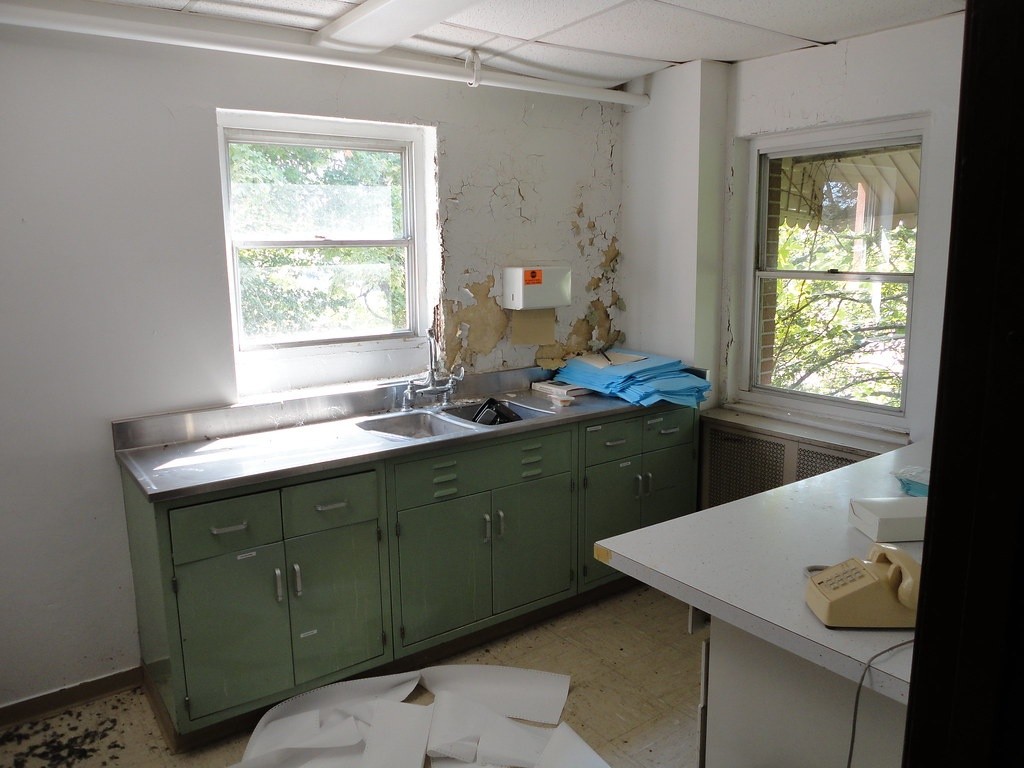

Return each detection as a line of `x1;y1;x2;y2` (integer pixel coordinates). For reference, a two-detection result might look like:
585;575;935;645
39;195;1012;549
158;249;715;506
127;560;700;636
532;380;594;396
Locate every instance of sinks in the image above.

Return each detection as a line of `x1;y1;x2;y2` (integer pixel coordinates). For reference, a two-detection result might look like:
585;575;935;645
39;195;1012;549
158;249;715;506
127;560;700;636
354;409;478;445
434;399;558;429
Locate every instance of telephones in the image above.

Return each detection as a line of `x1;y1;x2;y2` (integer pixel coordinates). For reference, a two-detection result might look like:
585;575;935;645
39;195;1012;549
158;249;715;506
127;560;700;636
803;542;921;630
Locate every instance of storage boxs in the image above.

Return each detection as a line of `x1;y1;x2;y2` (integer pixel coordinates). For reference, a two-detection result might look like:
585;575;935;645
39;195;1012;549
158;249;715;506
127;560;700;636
849;496;927;542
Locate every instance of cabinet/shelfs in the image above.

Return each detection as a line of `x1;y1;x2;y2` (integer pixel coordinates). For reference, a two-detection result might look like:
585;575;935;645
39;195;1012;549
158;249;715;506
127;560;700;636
105;404;907;767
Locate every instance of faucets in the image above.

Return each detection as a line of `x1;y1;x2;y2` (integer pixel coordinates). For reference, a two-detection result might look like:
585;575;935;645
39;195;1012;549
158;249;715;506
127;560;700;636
428;337;440;388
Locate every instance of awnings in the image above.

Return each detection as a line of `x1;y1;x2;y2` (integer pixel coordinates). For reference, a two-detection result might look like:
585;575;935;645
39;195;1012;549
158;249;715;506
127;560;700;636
779;148;920;232
